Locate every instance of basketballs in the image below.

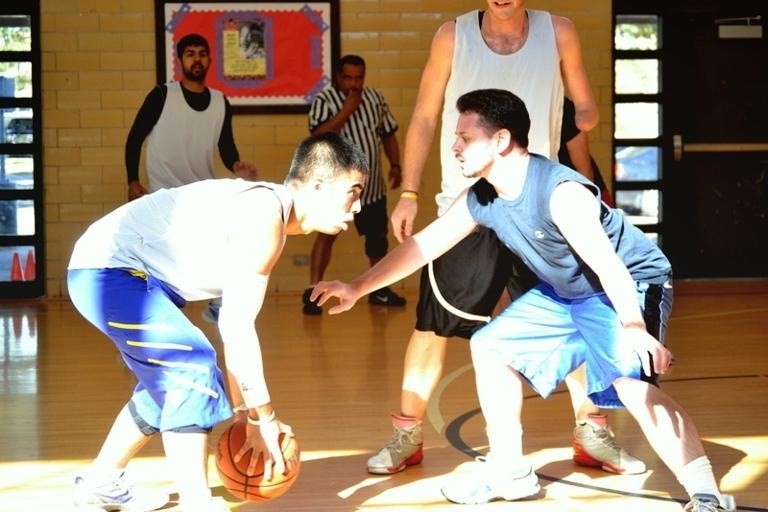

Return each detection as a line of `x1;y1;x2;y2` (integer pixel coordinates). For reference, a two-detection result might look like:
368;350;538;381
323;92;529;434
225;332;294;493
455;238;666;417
216;417;300;502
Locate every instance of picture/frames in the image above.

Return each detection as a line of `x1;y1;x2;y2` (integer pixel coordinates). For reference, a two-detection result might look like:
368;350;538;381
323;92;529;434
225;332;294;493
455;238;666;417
154;1;338;116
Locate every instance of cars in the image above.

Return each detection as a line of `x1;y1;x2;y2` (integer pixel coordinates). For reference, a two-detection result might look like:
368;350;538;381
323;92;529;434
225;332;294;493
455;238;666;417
614;146;660;216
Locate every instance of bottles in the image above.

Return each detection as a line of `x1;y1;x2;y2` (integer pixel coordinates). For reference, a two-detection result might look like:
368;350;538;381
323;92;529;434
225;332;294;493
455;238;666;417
10;252;23;282
23;250;37;281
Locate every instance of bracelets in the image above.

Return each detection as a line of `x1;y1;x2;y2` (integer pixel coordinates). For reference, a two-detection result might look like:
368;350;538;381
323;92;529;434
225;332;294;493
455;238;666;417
401;189;420;200
245;409;278;425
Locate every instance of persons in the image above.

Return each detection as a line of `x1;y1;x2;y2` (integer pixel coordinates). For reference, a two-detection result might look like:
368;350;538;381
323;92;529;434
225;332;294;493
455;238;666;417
309;89;736;512
300;56;407;314
559;94;615;208
60;131;370;512
125;33;257;325
365;0;647;476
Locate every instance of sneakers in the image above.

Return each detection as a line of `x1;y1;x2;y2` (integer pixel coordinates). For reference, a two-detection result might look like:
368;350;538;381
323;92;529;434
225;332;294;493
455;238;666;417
369;289;407;306
441;453;541;504
572;415;646;474
201;300;222;324
685;494;736;511
367;413;422;474
302;288;323;314
71;471;170;512
